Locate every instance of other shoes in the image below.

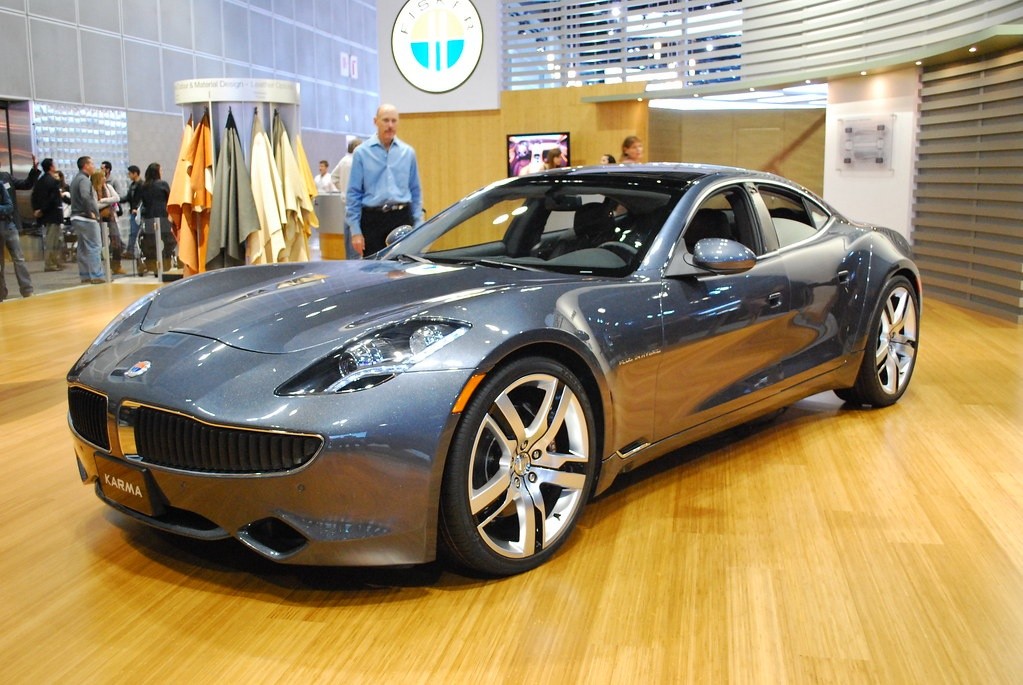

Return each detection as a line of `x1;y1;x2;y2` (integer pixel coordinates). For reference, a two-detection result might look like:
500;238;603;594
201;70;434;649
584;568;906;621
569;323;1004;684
0;296;3;302
20;287;33;298
91;278;113;284
81;278;91;284
44;263;67;272
120;252;134;260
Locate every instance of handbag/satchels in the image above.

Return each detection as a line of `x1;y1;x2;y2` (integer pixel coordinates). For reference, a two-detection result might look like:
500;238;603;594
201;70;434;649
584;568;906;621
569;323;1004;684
106;183;123;216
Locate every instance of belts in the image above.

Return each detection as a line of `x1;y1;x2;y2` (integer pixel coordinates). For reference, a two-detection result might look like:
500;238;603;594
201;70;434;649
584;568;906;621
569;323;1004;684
363;203;408;213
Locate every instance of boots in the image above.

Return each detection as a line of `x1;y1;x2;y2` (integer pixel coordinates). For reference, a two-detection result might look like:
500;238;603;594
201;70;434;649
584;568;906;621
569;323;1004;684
112;259;128;275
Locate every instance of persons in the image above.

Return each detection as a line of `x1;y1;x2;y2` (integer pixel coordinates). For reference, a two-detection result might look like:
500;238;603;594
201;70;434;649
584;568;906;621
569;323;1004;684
140;162;170;231
54;171;72;225
539;148;562;171
117;165;145;259
344;104;422;260
312;160;337;193
70;156;113;283
331;139;364;261
0;154;42;301
600;155;615;164
620;136;643;163
90;160;127;274
30;158;64;271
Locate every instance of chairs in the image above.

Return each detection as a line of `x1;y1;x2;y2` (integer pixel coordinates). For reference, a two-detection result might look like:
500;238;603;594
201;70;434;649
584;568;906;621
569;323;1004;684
547;199;619;261
684;207;735;255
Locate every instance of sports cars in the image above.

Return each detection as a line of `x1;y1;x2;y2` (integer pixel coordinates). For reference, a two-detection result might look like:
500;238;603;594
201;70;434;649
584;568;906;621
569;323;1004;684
65;160;925;581
